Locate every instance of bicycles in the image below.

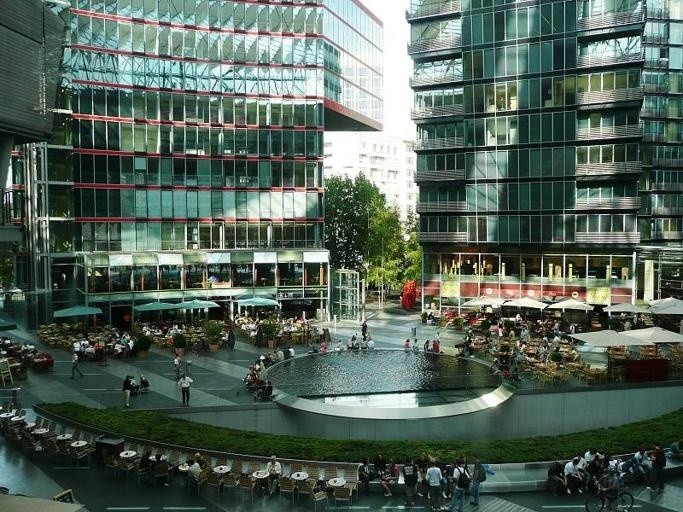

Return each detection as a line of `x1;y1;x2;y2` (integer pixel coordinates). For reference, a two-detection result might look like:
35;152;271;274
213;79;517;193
585;476;634;512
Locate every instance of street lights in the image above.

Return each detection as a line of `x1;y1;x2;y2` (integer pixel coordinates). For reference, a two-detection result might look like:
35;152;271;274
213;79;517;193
364;204;371;298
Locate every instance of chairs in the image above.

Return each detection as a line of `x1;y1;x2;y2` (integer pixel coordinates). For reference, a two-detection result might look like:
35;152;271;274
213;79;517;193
0;311;328;385
1;410;364;510
460;311;682;389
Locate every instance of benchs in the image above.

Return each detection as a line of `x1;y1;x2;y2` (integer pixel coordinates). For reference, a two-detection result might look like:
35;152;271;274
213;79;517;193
366;443;682;510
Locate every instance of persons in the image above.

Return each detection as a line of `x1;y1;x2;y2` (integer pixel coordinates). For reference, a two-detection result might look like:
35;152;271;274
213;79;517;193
0;309;683;410
176;446;668;511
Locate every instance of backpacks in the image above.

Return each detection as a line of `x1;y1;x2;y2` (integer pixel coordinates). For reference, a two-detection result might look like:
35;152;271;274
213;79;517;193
457;467;469;488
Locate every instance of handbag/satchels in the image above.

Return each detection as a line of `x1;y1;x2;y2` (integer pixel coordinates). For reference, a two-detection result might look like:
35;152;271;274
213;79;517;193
404;474;417;487
477;466;486;482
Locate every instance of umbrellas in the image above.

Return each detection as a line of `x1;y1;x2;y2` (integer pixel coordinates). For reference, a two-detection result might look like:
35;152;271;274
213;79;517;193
239;296;277;306
233;292;273;299
52;304;103;319
175;298;220;310
460;293;683;347
130;301;181;312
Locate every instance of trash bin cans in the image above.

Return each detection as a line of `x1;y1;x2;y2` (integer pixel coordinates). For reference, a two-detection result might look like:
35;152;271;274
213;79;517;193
411;327;416;336
